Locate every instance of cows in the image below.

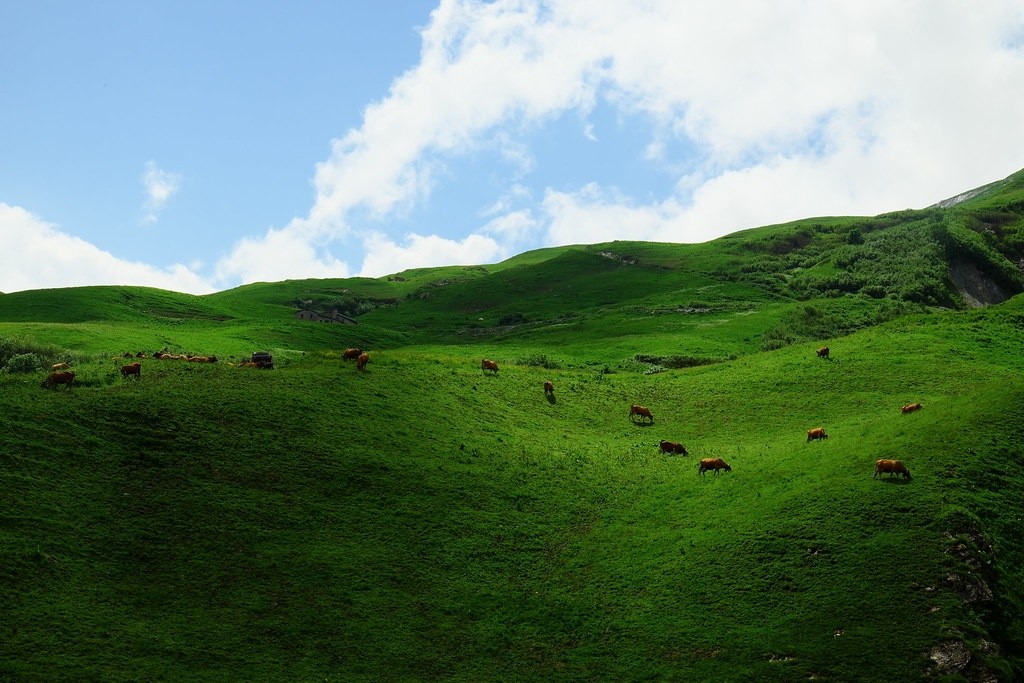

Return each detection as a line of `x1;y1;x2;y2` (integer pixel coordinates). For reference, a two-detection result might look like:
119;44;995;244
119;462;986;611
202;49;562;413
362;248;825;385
41;364;75;391
807;427;827;441
153;351;218;363
628;404;653;423
120;362;141;379
239;361;259;369
815;347;829;358
873;459;913;481
544;382;554;395
341;348;369;370
659;440;689;457
901;403;922;413
481;359;500;375
699;458;731;475
